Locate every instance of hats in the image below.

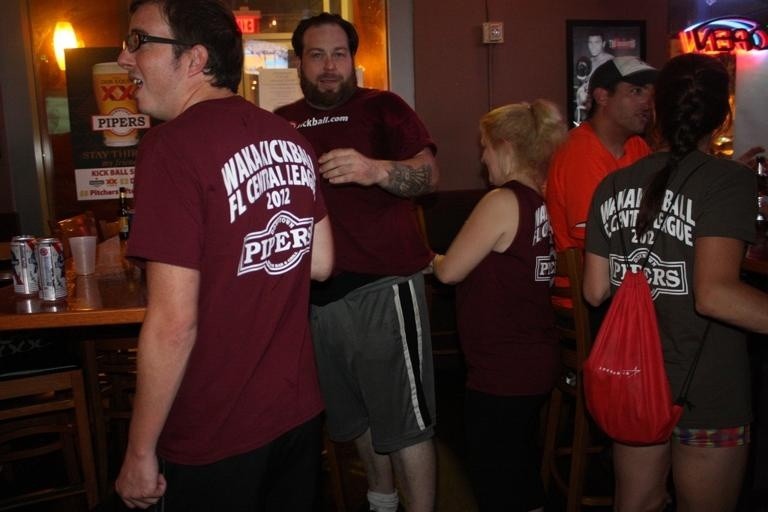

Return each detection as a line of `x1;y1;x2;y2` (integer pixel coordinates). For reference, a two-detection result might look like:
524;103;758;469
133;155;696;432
590;57;662;88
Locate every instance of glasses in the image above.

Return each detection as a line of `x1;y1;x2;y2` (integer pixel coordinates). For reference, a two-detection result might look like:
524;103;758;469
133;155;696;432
123;33;195;52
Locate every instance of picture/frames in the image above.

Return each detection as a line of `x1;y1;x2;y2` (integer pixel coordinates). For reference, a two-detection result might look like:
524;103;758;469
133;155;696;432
566;19;646;130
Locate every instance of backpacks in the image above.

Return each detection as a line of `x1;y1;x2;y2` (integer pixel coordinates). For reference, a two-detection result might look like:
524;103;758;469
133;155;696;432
582;154;709;445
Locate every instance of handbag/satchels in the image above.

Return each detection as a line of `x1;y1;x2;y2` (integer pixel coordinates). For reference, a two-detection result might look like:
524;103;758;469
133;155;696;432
309;269;383;307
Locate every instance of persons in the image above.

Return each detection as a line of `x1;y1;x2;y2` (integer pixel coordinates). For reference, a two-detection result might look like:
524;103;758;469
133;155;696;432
114;1;335;512
581;53;768;512
546;54;662;512
273;13;441;512
432;97;563;511
573;31;615;122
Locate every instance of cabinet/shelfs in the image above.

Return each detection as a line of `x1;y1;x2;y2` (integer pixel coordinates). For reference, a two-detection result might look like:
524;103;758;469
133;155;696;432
238;33;303;112
19;0;163;237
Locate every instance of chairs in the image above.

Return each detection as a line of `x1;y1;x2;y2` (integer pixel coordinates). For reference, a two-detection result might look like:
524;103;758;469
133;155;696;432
538;247;619;511
0;370;99;511
319;418;347;510
80;330;141;504
413;204;476;425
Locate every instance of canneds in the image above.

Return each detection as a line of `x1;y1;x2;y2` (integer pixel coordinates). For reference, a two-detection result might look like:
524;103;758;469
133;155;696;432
36;237;68;304
9;235;39;300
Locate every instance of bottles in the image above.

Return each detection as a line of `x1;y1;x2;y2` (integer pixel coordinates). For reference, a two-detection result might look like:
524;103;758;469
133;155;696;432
118;240;136;292
116;186;131;240
754;156;768;231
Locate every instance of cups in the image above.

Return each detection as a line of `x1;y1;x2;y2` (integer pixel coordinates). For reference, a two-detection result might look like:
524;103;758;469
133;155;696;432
69;236;96;275
76;277;103;311
93;62;142;148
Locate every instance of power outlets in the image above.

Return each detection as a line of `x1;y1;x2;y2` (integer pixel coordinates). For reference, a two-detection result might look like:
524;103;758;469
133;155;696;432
482;22;504;44
